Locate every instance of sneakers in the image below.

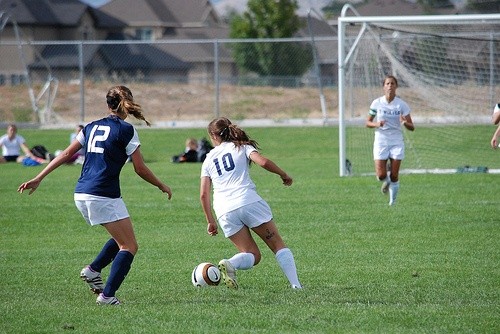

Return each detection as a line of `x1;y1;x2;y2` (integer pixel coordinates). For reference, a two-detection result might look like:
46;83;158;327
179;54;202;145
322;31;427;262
95;293;122;305
80;265;105;295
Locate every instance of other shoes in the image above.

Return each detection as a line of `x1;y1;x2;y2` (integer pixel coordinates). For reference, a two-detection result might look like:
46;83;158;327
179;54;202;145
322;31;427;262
382;172;391;194
290;284;303;290
218;259;239;291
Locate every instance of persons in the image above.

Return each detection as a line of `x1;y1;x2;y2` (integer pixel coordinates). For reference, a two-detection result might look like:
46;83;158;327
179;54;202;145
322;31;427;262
0;124;50;164
491;125;500;150
366;75;414;206
492;103;500;124
184;138;201;154
66;124;85;164
200;117;302;288
16;85;171;305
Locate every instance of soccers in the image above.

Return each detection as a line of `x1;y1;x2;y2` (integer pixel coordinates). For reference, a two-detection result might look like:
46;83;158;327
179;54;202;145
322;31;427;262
190;262;222;288
